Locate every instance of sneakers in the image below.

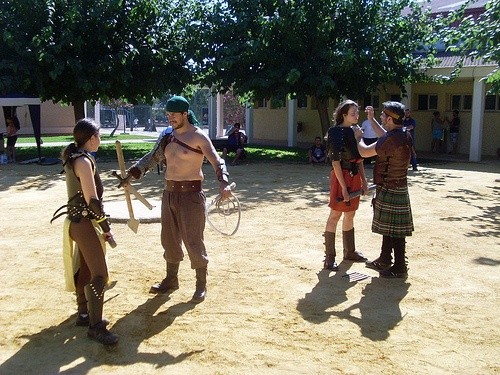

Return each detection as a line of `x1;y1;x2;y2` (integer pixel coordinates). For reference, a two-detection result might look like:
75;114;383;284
77;314;89;326
87;321;120;344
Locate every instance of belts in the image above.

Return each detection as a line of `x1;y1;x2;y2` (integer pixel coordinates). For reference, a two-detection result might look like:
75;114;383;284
167;180;202;192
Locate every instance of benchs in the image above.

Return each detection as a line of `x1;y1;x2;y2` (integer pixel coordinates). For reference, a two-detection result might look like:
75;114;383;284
211;139;247;163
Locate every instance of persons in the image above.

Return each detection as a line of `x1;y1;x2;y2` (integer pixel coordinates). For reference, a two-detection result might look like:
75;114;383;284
118;96;232;303
134;116;139;130
430;111;446;154
445;110;460;155
222;122;248;166
2;116;18;163
403;108;418;171
353;101;415;279
322;99;368;271
361;112;379;168
308;136;328;167
63;117;120;345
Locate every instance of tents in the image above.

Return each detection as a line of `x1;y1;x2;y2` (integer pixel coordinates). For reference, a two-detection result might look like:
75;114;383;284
0;98;43;164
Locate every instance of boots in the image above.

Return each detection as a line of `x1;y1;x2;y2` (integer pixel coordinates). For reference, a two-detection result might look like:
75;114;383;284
150;262;179;292
322;231;340;271
342;227;367;262
379;238;409;278
193;269;207;302
366;235;394;272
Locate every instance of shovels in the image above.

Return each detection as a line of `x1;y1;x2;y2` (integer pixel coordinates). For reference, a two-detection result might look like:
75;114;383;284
115;140;140;234
